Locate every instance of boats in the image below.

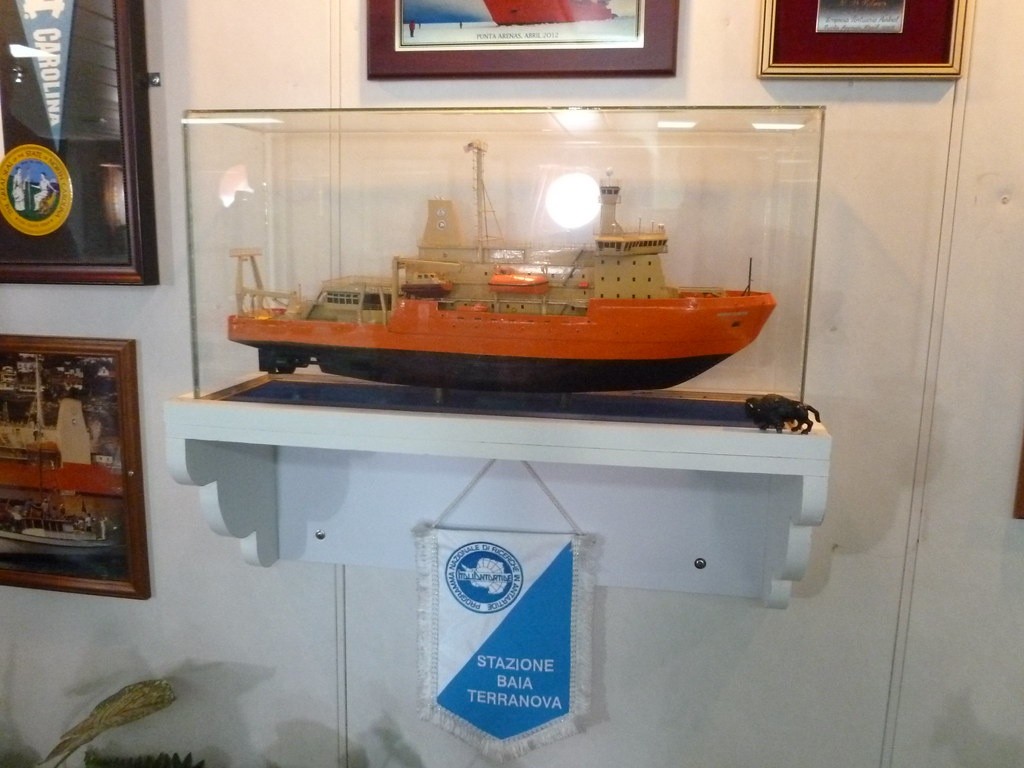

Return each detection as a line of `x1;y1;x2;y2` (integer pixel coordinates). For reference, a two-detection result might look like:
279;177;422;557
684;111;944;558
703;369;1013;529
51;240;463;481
0;393;127;527
228;134;778;396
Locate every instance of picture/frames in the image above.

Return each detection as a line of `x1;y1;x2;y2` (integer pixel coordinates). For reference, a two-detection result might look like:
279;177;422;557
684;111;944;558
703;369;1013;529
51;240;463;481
0;334;152;600
367;0;680;79
757;0;976;81
0;0;161;287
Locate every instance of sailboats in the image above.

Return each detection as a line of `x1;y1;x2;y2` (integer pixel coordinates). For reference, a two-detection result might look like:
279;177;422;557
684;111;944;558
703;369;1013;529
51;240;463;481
0;351;120;558
